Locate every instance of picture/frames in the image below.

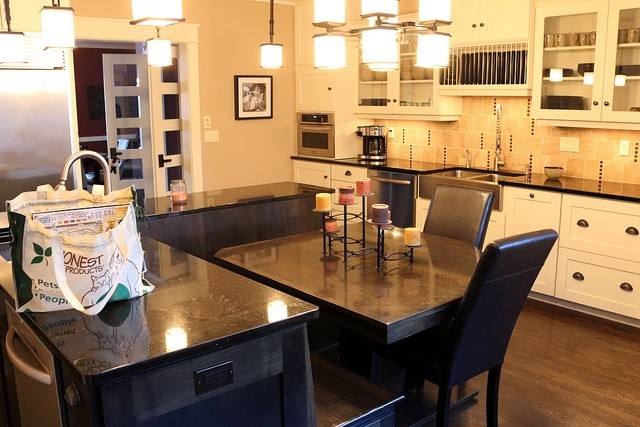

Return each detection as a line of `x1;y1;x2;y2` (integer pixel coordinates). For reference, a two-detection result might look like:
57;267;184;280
234;75;273;120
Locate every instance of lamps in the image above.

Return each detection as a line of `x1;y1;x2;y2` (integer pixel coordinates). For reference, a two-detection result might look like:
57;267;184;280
39;0;76;53
311;0;452;71
259;0;283;68
0;0;28;66
129;0;186;67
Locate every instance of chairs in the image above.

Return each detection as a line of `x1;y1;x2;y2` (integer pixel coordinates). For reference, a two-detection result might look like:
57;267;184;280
414;229;559;427
422;184;495;252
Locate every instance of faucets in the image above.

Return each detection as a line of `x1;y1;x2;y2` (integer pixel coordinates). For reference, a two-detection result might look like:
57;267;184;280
464;148;472;168
494;147;506;172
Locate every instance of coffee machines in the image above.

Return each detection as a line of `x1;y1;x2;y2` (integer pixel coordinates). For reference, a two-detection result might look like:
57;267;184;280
356;125;387;168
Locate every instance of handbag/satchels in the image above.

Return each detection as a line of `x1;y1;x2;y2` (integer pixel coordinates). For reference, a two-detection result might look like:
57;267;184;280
5;150;156;317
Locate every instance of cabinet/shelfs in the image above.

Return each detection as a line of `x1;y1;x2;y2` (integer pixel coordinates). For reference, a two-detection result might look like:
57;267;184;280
450;0;535;47
505;186;561;297
555;193;640;320
294;0;353;111
330;163;369;226
530;0;640;123
352;12;462;121
293;160;330;188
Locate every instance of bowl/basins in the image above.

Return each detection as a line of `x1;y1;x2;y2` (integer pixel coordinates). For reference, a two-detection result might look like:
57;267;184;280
361;64;387;81
401;61;433;80
544;166;564;180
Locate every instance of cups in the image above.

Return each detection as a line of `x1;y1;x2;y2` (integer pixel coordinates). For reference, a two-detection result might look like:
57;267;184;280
544;33;556;47
579;33;588;45
589;31;597;45
618;28;627;44
558;34;566;45
171;180;186;202
627;29;639;43
570;33;578;46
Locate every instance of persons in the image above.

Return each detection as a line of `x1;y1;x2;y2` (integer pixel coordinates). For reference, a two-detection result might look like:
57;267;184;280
250;83;266;111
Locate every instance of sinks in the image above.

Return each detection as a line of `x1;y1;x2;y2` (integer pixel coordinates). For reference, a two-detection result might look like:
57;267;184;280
431;170;485;178
472;174;514;185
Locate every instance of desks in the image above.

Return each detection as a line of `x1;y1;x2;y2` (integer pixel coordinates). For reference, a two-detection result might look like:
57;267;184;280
215;220;482;427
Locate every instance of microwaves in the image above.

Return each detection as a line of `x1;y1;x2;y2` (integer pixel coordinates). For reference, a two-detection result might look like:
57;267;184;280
298;113;335;159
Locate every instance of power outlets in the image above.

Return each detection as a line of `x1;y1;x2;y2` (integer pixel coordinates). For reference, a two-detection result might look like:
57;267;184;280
388;127;394;138
194;360;234;396
204;116;211;128
